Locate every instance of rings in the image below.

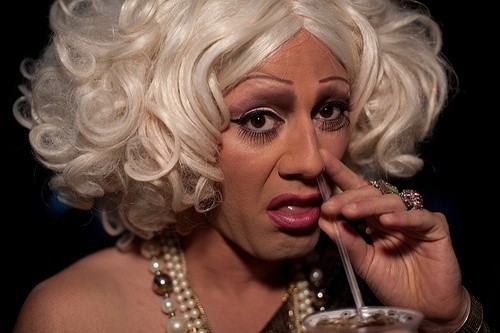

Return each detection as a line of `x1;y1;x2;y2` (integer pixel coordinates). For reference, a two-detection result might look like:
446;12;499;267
368;178;424;210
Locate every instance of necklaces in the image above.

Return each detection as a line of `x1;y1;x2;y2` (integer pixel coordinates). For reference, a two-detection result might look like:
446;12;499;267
139;224;331;332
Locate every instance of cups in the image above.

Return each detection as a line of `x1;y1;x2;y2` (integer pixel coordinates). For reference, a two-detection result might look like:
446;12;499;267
303;305;426;333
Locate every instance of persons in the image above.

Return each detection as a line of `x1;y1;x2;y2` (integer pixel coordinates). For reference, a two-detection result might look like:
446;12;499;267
11;0;499;333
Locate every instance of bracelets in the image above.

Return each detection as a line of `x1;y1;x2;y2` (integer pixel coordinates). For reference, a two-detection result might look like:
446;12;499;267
448;283;499;333
